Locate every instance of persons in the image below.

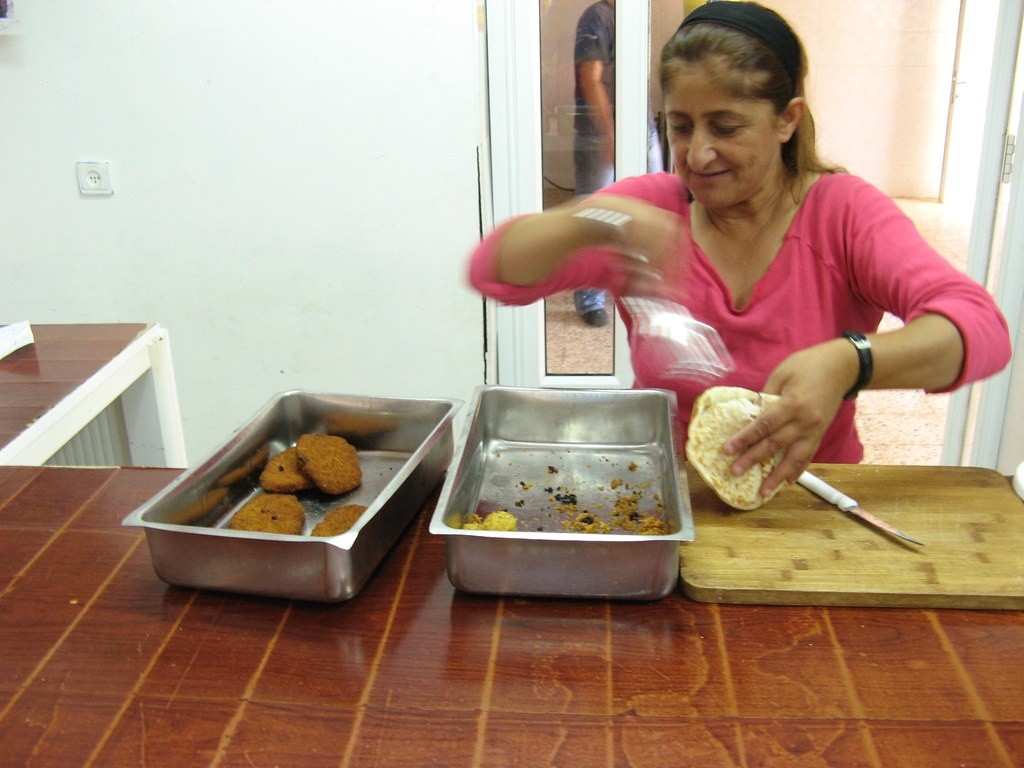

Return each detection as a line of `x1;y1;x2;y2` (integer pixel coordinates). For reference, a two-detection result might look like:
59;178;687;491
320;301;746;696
465;0;1012;497
573;0;616;328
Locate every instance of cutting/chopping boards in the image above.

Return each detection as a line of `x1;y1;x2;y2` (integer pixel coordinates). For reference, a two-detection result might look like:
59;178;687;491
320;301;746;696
679;460;1024;609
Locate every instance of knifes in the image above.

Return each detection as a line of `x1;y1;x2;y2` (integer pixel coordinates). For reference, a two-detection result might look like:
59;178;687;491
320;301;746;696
798;471;924;546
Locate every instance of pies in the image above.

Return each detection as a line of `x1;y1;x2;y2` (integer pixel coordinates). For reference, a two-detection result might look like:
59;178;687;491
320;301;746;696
684;385;790;510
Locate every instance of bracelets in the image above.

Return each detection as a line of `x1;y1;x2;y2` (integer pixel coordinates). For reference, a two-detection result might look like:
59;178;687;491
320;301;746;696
841;330;874;401
